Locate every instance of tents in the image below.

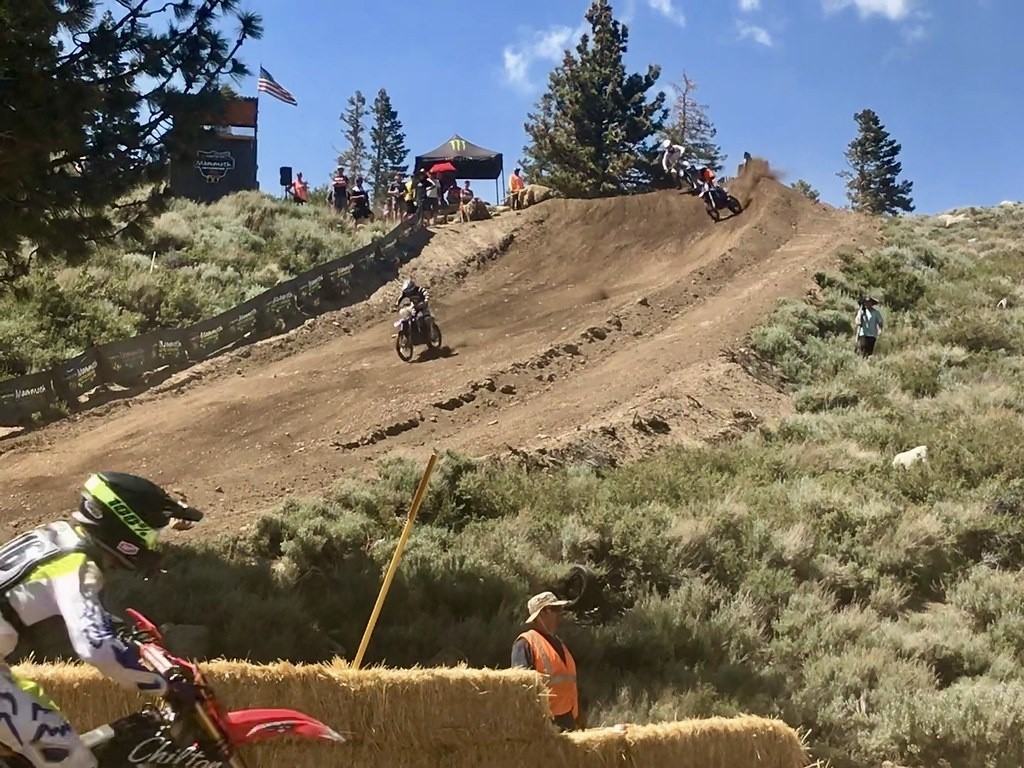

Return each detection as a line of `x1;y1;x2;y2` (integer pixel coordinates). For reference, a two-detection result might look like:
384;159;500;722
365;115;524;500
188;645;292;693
412;133;506;206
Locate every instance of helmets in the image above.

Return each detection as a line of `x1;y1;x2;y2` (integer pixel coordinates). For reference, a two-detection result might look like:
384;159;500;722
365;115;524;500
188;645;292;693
72;471;204;570
401;281;416;297
663;139;673;152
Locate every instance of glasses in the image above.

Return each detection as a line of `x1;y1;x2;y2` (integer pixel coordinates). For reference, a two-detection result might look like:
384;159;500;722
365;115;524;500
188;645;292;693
542;605;561;613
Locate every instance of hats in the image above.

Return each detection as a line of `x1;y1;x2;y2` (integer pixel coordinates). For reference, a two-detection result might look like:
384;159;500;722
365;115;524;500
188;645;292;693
525;592;569;623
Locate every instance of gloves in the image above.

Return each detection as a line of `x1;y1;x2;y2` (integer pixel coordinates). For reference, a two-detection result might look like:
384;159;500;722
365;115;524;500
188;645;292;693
107;613;129;642
160;667;189;703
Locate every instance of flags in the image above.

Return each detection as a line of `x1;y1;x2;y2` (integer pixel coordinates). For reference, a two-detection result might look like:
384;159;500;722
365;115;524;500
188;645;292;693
257;66;298;107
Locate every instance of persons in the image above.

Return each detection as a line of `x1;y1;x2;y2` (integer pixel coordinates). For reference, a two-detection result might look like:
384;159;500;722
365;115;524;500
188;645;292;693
509;167;525;210
395;280;433;351
662;139;753;210
383;168;474;229
0;471;196;768
510;591;579;732
855;297;883;359
287;171;309;205
326;165;375;228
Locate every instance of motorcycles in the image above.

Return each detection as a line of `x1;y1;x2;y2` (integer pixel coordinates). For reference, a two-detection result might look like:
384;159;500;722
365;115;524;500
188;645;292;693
390;299;443;361
665;156;706;196
698;181;742;223
0;607;347;768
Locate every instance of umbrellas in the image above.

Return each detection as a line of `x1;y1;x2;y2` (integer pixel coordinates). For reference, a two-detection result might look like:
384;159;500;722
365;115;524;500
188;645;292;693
429;162;456;173
